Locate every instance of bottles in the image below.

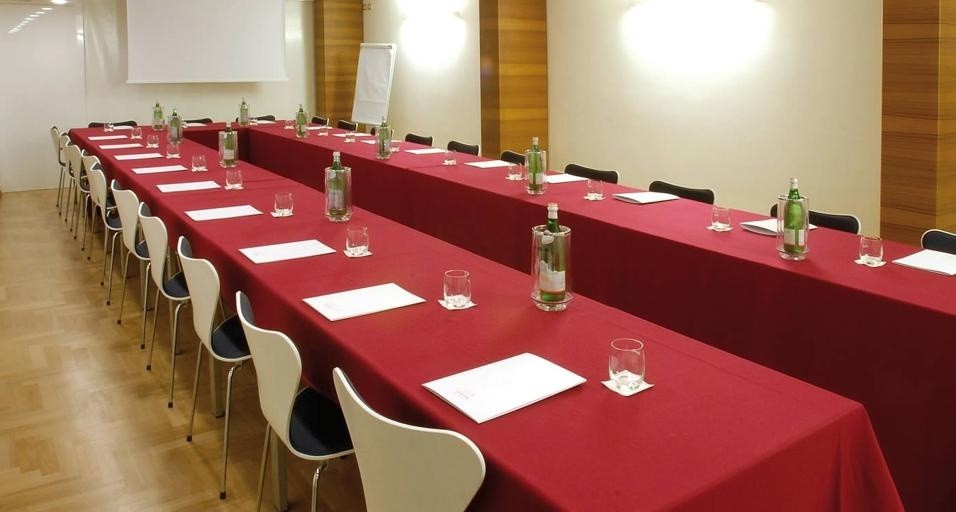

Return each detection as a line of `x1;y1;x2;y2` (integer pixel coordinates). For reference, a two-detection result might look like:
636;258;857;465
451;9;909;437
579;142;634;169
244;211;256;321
239;96;248;125
153;99;162;129
222;120;235;166
784;177;805;254
378;116;390;157
296;103;306;136
328;151;347;217
170;104;179;142
537;202;565;301
527;135;544;190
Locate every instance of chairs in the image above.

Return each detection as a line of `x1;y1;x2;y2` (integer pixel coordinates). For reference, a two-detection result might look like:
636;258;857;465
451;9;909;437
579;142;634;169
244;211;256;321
138;202;226;408
447;141;479;156
920;229;956;255
649;180;714;205
565;164;618;184
310;116;330;126
111;179;151;350
370;127;395;137
51;127;123;286
405;134;432;146
337;120;359;133
177;236;252;500
332;367;486;512
770;204;862;235
236;291;355;512
501;150;526;166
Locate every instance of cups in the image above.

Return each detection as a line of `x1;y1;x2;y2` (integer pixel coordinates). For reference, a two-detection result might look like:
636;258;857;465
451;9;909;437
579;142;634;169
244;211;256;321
166;114;183;144
345;226;370;256
344;129;356;143
608;338;646;389
443;269;472;307
237;104;250;127
250;116;257;126
323;166;353;223
524;149;547;195
146;134;159;147
151;106;165;132
131;127;143;137
295;111;309;138
442;147;457;165
218;130;239;168
858;234;883;265
374;126;391;160
191;151;206;171
711;205;730;229
273;191;294;215
224;167;243;188
391;139;401;152
587;177;603;201
317;123;328;136
529;224;576;312
165;138;182;158
776;192;810;261
507;161;522;179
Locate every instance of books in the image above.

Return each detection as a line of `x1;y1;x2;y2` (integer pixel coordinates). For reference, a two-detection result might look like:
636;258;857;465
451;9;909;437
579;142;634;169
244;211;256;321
464;160;517;169
360;140;402;144
239;240;336;265
546;174;588;183
740;219;818;235
257;120;276;124
306;126;333;130
423;351;586;424
185;204;262;221
113;153;164;160
404;148;448;154
332;132;372;137
114;125;133;130
156;181;222;192
88;135;128;141
891;249;956;276
187;123;207;126
301;283;427;322
131;165;188;174
611;192;680;204
97;143;143;149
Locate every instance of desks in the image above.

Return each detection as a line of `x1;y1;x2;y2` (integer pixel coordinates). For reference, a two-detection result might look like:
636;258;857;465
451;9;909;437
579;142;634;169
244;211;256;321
68;121;906;512
245;120;956;512
